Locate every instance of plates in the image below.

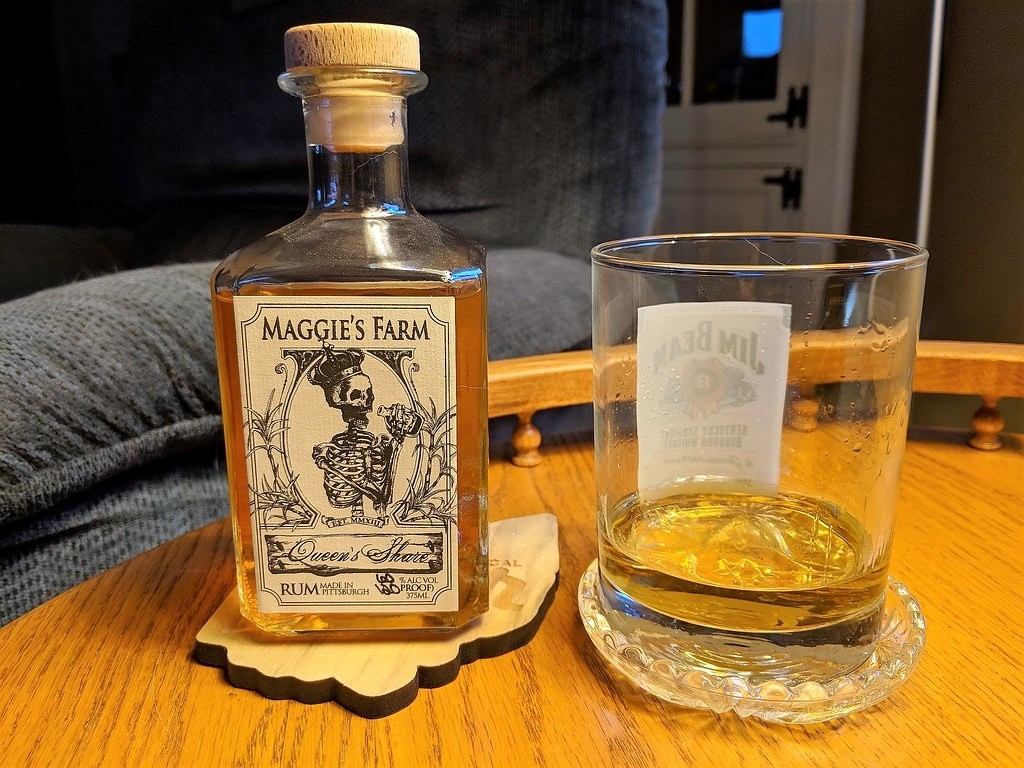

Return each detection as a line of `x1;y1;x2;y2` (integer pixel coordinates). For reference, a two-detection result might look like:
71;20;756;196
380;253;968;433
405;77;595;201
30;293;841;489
577;557;925;723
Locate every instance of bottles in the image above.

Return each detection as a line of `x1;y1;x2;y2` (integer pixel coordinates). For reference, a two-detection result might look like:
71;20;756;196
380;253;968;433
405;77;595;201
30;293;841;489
210;21;490;644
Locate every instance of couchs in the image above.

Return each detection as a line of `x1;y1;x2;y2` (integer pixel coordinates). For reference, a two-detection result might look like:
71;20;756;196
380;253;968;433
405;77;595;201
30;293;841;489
0;0;668;629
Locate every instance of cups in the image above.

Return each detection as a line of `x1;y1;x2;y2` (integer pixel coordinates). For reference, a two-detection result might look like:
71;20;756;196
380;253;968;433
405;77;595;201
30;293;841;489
591;230;930;683
